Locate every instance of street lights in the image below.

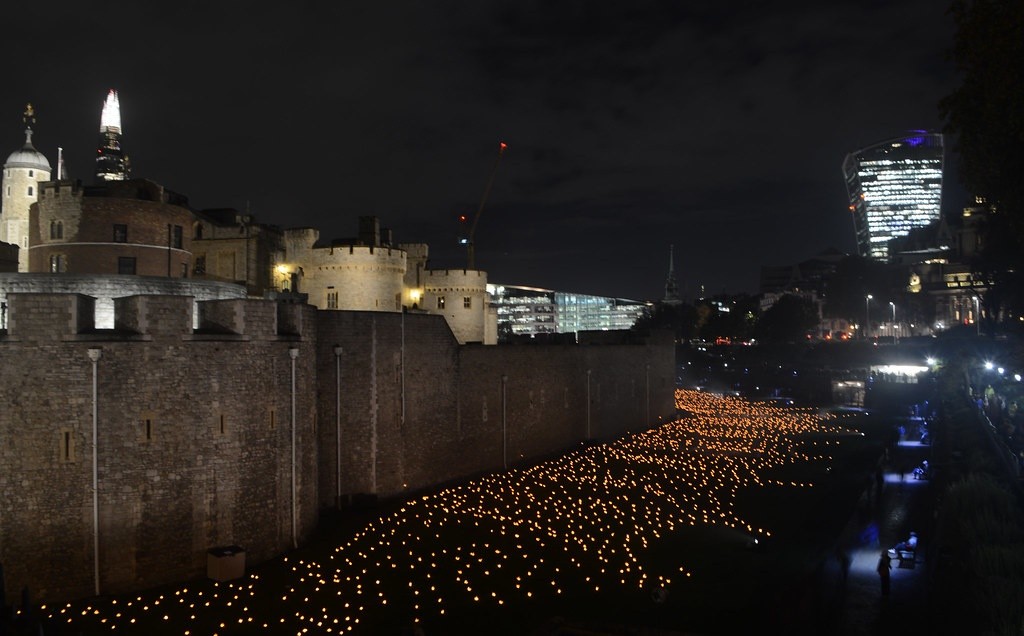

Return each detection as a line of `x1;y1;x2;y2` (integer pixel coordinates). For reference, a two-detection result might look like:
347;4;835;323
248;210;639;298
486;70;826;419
890;302;896;345
973;297;979;333
866;295;872;312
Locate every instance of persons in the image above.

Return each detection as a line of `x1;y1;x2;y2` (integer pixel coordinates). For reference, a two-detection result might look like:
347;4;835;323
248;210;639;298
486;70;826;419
889;531;918;559
870;369;908;384
897;425;905;441
913;399;929;418
877;549;892;595
919;422;927;443
914;460;929;480
969;383;1024;469
865;521;880;548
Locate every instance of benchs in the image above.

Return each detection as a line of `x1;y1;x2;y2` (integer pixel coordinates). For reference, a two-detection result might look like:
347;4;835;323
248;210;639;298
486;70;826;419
898;538;920;565
919;469;929;480
